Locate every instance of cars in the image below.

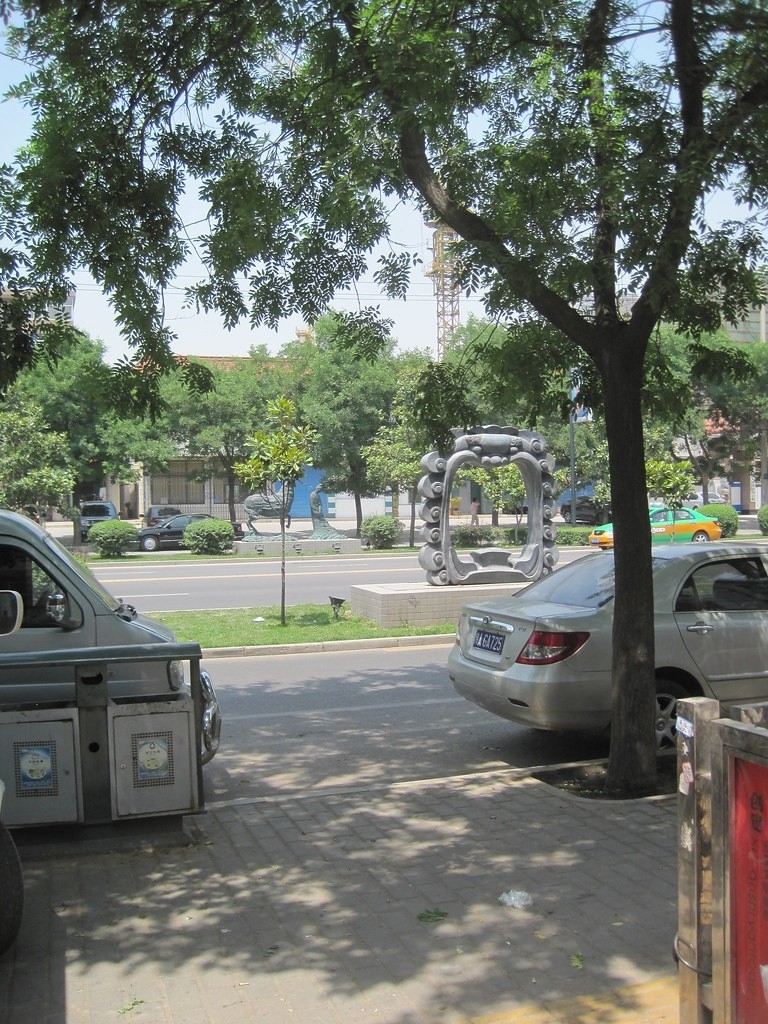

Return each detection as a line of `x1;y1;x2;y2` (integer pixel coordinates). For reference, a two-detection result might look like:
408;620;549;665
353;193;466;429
560;495;613;525
516;502;529;515
126;514;244;552
588;502;722;550
444;541;768;749
21;505;46;526
679;492;726;511
139;506;182;528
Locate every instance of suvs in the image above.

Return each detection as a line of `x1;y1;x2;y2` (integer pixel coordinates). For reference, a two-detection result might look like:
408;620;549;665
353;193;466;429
79;500;124;543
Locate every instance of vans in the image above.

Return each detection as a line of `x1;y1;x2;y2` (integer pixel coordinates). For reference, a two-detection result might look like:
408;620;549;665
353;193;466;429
0;509;222;769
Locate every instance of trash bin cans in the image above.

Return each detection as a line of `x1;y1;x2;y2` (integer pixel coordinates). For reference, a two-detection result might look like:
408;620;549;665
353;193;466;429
0;641;208;843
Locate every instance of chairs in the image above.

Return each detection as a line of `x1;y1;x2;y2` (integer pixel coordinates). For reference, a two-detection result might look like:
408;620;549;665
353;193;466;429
712;579;754;602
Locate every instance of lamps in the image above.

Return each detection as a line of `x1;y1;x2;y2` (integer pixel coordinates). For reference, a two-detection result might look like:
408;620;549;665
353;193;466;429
329;596;346;609
